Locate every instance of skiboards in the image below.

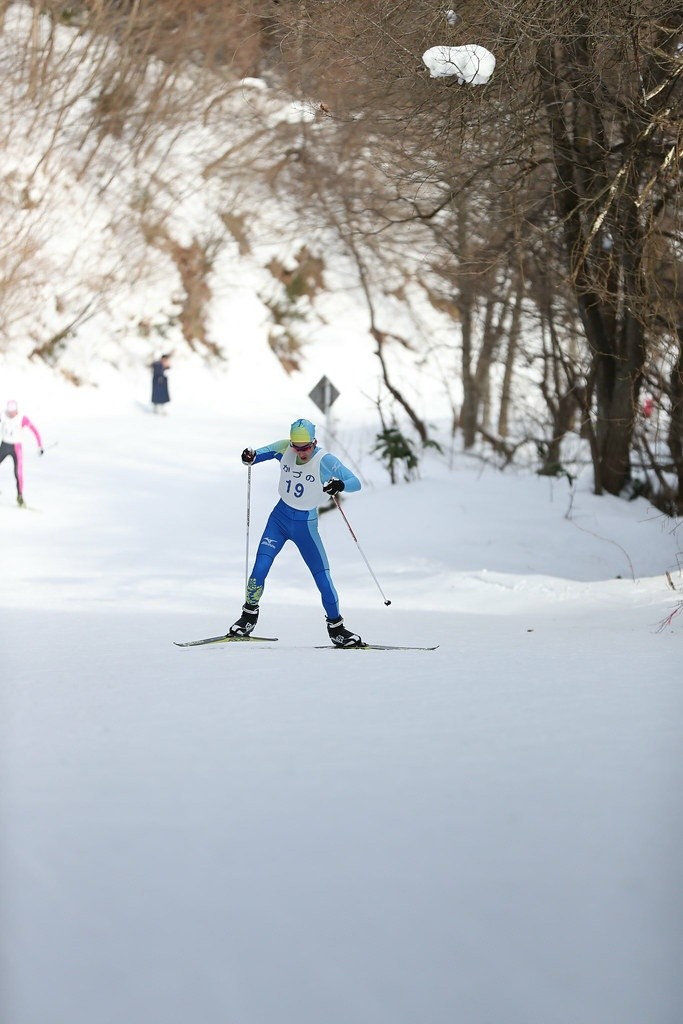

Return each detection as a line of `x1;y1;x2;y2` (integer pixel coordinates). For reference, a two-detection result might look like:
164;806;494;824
171;634;441;650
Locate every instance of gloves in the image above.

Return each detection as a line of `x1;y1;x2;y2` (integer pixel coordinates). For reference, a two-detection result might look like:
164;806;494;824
323;479;345;496
241;448;257;464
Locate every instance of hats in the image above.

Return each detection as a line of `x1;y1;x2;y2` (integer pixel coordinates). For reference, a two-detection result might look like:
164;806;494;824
289;419;316;443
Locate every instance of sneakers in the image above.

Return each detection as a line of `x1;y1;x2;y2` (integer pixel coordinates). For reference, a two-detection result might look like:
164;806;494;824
325;615;366;648
227;604;259;637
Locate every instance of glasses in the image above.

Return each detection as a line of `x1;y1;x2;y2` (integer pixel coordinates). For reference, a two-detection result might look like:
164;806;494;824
289;444;312;452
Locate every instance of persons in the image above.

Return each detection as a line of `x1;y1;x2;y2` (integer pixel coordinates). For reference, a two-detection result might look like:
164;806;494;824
146;354;169;415
230;419;361;648
0;400;44;505
